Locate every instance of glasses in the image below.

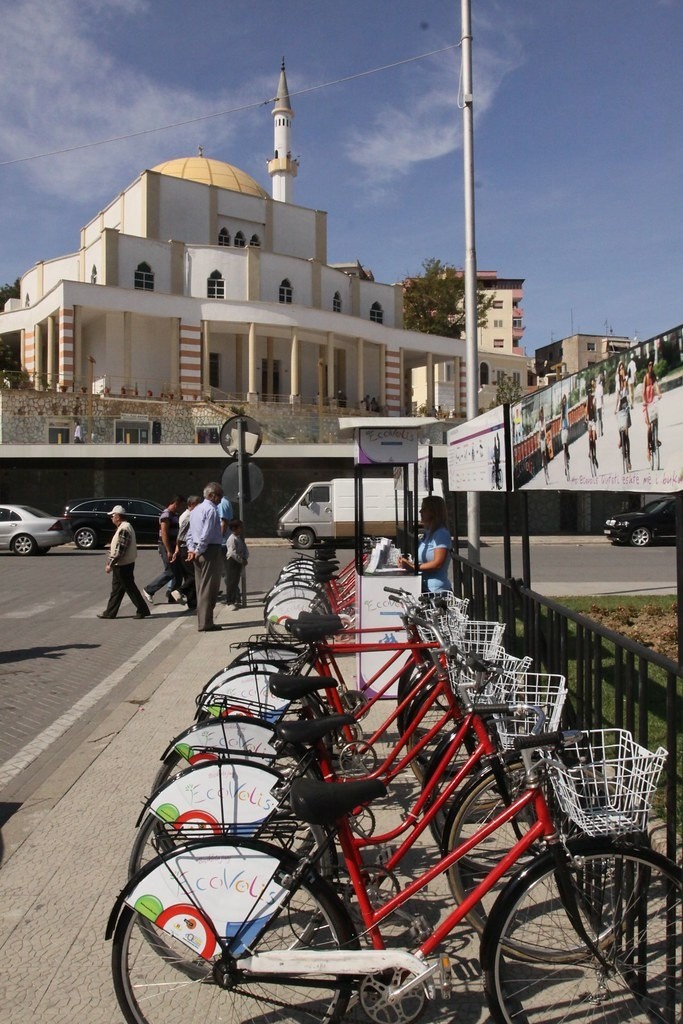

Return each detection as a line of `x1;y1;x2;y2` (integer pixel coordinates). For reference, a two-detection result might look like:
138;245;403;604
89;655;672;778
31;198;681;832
211;492;223;498
648;363;654;366
111;515;117;518
619;367;625;371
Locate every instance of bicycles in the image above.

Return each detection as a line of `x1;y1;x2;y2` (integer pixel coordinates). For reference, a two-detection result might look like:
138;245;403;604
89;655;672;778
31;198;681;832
104;530;682;1023
538;395;661;486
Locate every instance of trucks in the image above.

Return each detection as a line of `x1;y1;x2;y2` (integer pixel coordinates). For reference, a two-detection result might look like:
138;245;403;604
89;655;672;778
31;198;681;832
276;476;451;550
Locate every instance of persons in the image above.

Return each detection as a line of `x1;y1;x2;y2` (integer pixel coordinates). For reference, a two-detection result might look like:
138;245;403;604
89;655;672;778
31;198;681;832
431;406;437;417
74;420;83;443
364;395;380;413
337;390;347;408
143;481;250;631
539;353;662;474
398;496;453;596
438;406;442;413
96;505;151;619
494;432;502;490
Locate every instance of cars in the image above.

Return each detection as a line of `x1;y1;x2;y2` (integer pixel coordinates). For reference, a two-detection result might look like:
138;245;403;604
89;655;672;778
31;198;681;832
603;493;681;547
0;504;74;557
64;497;180;552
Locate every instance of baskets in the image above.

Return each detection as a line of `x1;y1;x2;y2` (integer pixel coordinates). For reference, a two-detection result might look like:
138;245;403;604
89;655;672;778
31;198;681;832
540;442;545;451
489;672;568;750
537;729;668;837
388;543;404;568
617;409;628;432
560;430;569;443
411;590;507;662
587;420;595;431
647;399;661;422
453;639;532;707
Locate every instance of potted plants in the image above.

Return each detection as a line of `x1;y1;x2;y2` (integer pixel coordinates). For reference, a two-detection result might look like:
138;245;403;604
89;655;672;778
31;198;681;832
179;391;184;400
169;394;174;399
134;382;139;396
119;386;127;394
147;390;153;396
80;387;88;393
193;394;198;400
103;386;110;393
58;383;69;392
160;391;164;397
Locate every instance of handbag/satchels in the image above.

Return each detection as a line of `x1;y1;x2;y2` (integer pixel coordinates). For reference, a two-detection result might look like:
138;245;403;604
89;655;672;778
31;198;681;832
626;368;631;378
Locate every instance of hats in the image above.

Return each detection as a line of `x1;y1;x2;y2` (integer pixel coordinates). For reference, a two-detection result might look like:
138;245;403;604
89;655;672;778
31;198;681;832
107;505;125;515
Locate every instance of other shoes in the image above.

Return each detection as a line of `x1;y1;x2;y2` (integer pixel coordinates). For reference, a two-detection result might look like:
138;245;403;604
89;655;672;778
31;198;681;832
142;589;153;603
171;591;185;606
133;613;150;619
218;591;223;596
205;624;221;631
96;613;113;619
222;600;235;604
